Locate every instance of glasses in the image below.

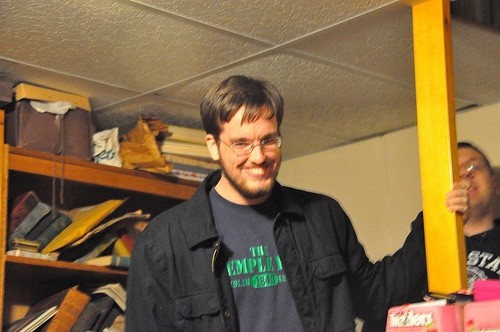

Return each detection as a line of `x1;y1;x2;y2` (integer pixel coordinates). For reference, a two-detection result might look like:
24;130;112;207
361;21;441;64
216;131;283;155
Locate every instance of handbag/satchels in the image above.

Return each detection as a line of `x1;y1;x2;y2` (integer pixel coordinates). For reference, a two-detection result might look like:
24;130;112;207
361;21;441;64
4;97;92;220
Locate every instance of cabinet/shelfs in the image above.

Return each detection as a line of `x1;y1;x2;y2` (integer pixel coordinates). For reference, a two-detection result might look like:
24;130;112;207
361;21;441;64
0;109;202;332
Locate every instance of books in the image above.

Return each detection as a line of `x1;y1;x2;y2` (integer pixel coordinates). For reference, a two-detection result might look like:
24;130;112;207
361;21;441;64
157;122;221;184
5;191;153;269
3;280;127;332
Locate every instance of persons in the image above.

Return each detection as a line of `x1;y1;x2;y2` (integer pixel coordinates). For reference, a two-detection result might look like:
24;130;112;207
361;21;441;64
456;142;500;295
126;74;471;332
